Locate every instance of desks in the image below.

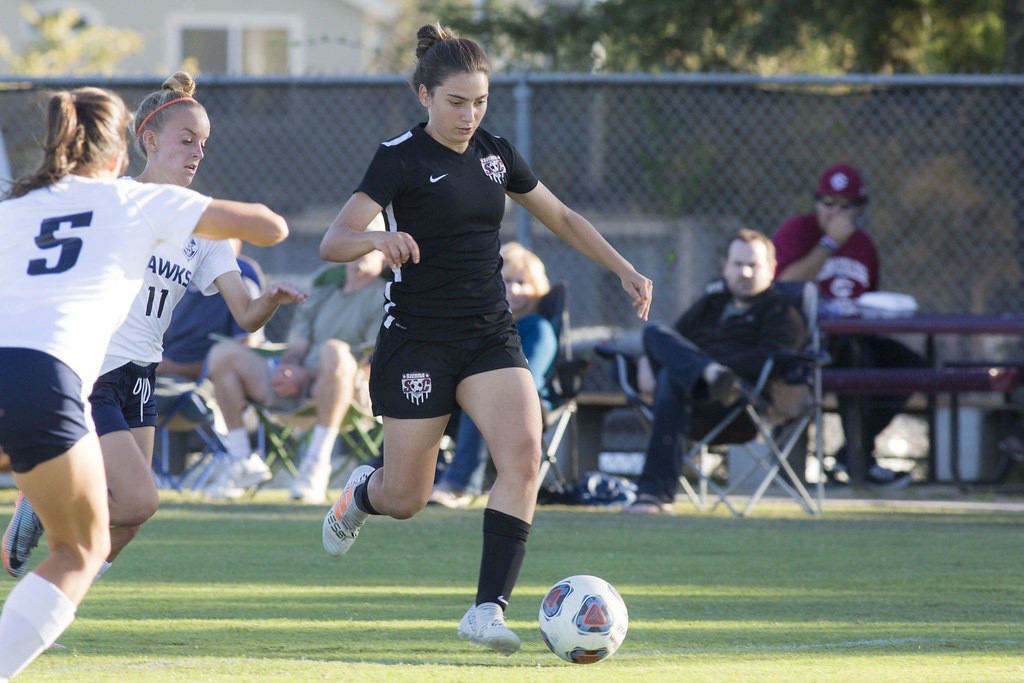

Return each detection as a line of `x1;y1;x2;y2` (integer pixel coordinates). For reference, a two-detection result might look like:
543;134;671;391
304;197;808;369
820;316;1023;489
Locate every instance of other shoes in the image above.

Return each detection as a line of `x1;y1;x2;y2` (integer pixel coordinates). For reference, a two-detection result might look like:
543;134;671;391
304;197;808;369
714;371;742;407
427;482;473;510
627;495;673;516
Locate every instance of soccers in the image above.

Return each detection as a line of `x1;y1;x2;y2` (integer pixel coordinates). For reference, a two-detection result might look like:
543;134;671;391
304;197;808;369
538;575;629;664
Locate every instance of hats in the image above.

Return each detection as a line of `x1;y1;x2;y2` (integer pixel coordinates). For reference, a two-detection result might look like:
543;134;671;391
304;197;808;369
817;163;868;199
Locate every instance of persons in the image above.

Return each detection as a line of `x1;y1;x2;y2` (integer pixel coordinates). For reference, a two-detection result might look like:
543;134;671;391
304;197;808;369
625;227;807;514
429;241;559;506
206;213;387;506
156;238;262;380
0;73;309;647
772;165;921;488
0;86;290;683
320;22;653;658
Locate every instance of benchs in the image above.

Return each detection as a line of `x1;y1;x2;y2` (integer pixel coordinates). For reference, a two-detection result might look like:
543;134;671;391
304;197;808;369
812;368;1024;493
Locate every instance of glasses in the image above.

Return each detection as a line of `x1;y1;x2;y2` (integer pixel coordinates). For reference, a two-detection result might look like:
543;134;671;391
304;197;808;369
823;194;851;210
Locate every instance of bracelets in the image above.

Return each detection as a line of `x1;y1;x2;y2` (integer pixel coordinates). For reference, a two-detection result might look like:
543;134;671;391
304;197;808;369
818;238;837;255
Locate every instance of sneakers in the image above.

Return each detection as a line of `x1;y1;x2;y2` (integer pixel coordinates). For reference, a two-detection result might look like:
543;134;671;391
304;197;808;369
1;489;47;577
321;465;376;557
836;447;876;471
291;455;332;502
203;453;273;495
866;466;911;492
458;602;520;656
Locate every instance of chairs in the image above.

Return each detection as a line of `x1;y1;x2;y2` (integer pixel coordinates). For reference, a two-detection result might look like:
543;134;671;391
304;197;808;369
145;337;820;522
